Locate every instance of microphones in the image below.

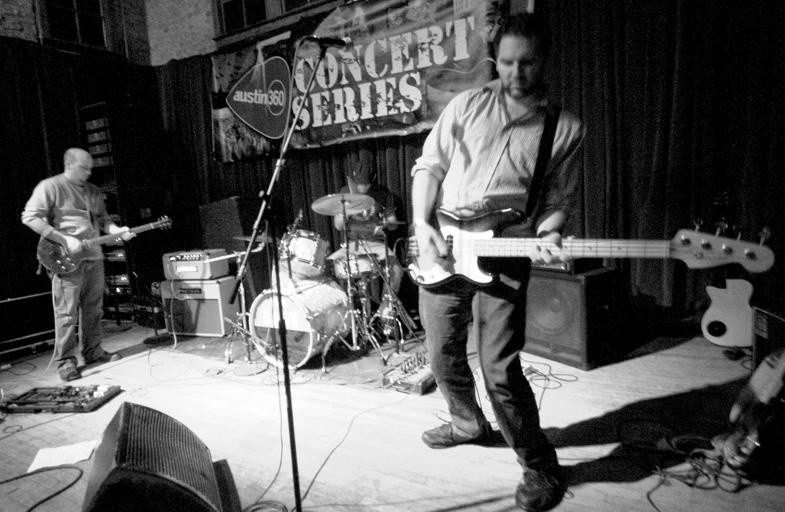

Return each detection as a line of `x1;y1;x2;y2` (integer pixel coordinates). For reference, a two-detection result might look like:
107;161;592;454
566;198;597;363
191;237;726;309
308;36;353;49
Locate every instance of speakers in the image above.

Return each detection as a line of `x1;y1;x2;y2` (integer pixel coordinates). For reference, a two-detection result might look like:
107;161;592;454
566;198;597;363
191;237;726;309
520;267;633;371
753;295;785;404
160;276;241;338
198;196;279;331
81;402;222;512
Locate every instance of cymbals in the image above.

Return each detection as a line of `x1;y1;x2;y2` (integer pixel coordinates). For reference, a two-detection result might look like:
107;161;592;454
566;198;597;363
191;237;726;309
312;193;372;215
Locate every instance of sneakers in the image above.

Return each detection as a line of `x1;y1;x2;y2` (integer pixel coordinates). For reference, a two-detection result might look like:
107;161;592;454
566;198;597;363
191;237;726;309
423;422;492;448
86;352;121;362
59;359;80;380
516;444;563;512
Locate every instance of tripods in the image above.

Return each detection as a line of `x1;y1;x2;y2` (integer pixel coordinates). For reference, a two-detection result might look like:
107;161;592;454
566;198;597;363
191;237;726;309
318;202;416;373
224;258;253;363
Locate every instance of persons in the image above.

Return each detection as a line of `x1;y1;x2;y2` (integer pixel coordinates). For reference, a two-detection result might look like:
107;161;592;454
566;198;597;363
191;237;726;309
334;161;406;321
20;146;136;382
409;15;588;510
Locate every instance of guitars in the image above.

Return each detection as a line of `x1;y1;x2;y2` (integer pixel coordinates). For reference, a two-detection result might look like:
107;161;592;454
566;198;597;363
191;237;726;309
700;191;760;348
405;207;774;289
36;215;173;274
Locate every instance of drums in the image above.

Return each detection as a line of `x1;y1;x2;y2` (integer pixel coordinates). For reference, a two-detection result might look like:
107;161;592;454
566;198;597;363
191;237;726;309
250;280;351;368
333;254;377;279
231;235;273;242
280;231;330;278
